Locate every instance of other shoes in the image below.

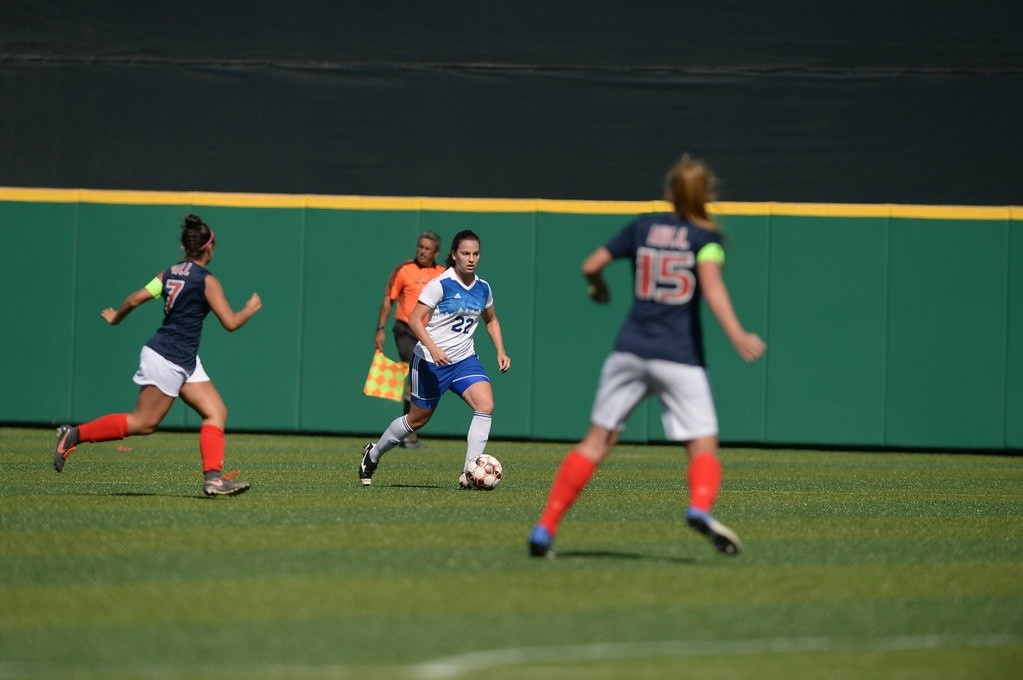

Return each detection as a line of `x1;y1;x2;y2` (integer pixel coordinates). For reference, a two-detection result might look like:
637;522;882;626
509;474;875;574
401;440;426;449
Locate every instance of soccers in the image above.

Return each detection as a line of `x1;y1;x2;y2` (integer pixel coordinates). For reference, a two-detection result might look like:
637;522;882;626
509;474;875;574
466;454;504;491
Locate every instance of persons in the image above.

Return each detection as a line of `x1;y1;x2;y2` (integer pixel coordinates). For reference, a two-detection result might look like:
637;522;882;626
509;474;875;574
358;230;511;487
527;159;767;558
55;215;264;497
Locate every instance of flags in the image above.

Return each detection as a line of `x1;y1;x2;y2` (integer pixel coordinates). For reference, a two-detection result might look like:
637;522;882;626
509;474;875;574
363;349;410;402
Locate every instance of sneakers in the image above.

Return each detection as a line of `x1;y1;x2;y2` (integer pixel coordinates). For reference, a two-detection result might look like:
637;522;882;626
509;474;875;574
203;476;250;496
359;442;379;487
459;473;471;489
529;526;552;560
53;425;76;472
687;509;742;557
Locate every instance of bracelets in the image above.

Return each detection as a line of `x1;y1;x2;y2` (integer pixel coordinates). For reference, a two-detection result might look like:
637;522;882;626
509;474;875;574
377;324;385;330
